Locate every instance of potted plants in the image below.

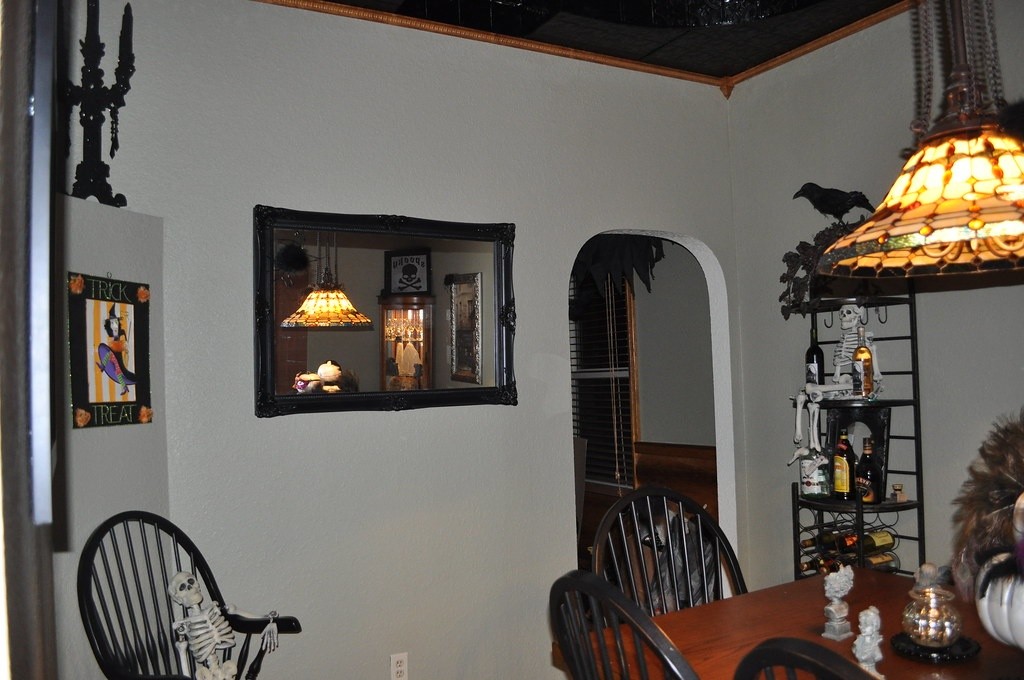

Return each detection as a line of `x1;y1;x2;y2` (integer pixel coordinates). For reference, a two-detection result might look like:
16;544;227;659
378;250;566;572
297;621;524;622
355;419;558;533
778;215;866;320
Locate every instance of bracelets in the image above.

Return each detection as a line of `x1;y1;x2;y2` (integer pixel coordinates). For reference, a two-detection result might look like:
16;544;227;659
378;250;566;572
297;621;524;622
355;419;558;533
1012;524;1024;534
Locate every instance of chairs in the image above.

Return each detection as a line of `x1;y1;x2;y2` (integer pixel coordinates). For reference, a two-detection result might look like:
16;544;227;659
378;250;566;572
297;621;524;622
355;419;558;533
734;637;879;680
550;488;749;680
77;510;302;680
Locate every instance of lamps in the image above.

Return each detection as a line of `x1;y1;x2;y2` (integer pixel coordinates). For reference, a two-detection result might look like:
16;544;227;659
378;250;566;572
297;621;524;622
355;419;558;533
279;231;373;327
813;0;1023;290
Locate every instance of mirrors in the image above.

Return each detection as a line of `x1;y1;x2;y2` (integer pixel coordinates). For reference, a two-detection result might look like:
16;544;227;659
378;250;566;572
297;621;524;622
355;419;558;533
252;203;517;418
444;272;482;384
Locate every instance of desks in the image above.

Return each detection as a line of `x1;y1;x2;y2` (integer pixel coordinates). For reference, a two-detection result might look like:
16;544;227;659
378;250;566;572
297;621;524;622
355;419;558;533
552;568;1024;680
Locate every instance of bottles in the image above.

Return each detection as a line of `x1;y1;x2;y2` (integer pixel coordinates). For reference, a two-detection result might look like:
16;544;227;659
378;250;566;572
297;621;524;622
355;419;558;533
806;328;825;385
799;445;831;500
803;355;804;356
800;530;899;575
852;326;874;396
855;437;884;504
833;429;855;501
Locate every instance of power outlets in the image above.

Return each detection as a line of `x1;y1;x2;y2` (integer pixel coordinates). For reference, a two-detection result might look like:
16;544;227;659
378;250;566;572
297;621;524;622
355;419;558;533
391;652;408;680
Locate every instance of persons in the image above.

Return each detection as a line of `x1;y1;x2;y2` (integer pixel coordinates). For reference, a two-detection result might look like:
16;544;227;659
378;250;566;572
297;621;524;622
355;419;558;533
823;564;854;620
851;609;884;665
949;421;1024;649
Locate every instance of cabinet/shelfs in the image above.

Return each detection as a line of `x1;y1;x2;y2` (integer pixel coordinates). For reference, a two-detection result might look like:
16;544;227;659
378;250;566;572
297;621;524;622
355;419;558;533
792;286;925;580
377;289;436;391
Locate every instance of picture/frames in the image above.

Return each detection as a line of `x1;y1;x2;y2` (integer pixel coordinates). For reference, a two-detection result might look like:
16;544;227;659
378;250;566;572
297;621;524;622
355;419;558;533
68;271;152;429
384;247;431;295
827;407;891;500
